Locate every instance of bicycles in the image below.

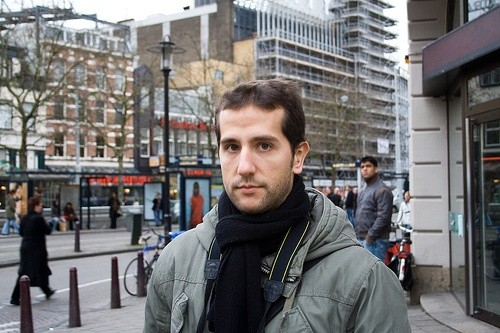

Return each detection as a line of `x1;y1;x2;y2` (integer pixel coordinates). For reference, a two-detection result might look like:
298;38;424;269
124;228;186;296
390;221;416;290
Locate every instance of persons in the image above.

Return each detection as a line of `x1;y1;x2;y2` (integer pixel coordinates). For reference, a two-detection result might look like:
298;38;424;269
343;185;355;231
326;186;334;202
9;195;57;306
50;198;66;232
121;193;133;206
333;187;342;207
144;80;412;333
395;191;413;240
189;180;204;229
63;201;82;231
353;187;359;219
151;192;163;227
355;156;393;267
2;191;20;236
107;191;122;229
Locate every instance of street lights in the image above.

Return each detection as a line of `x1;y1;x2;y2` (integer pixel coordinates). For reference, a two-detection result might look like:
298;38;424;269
147;34;187;248
